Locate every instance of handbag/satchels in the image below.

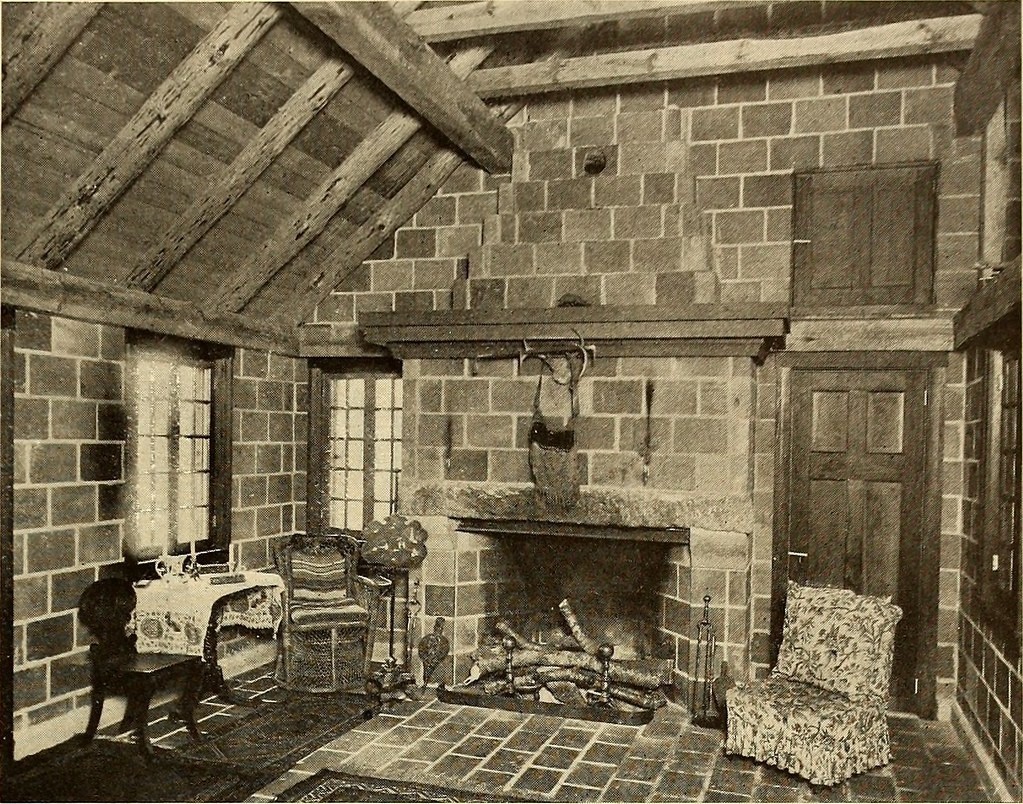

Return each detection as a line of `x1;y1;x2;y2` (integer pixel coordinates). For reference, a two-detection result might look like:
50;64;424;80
529;352;581;507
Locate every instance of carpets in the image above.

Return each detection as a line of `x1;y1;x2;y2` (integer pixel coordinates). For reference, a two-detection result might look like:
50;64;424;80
270;769;568;803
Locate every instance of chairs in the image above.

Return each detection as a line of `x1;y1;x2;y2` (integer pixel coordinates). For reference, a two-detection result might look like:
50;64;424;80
271;533;382;695
723;580;903;787
83;579;204;756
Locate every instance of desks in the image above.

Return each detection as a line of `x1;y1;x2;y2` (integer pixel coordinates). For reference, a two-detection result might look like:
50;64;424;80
134;574;291;716
